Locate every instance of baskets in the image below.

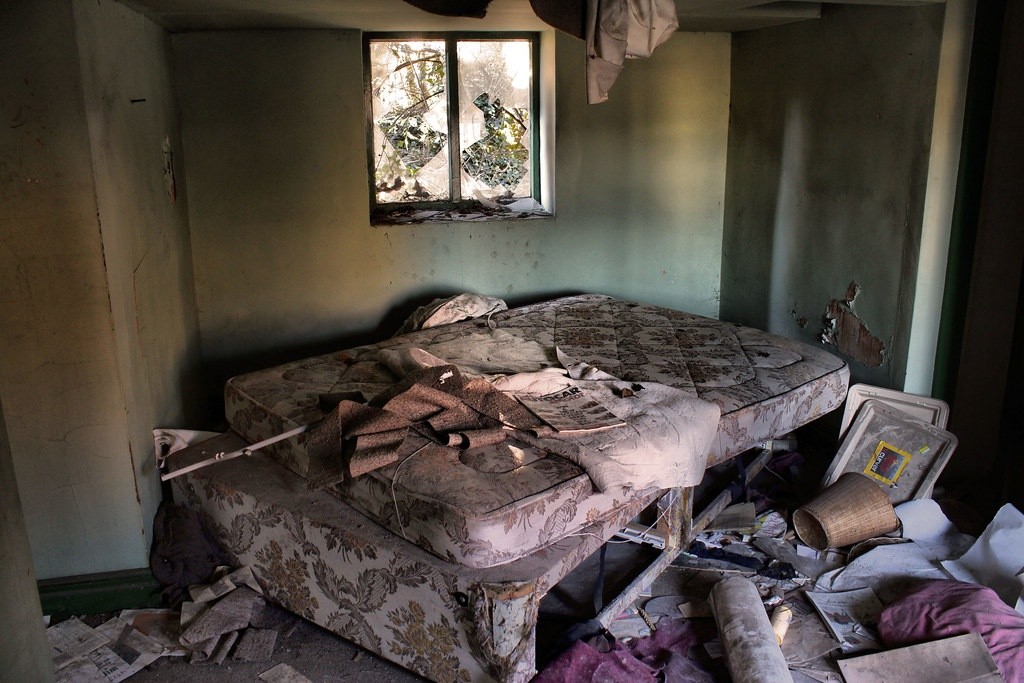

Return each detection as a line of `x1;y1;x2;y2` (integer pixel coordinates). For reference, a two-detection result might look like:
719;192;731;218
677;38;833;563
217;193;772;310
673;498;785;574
792;472;900;552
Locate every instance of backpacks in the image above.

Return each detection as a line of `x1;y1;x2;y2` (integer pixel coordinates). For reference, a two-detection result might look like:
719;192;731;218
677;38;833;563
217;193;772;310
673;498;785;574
152;503;220;587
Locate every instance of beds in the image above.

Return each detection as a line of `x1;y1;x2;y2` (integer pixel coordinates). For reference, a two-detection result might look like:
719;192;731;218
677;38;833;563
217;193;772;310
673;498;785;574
156;292;851;683
